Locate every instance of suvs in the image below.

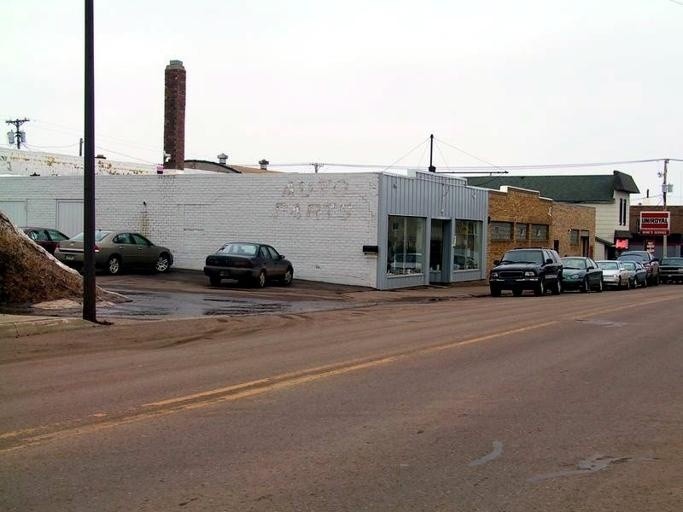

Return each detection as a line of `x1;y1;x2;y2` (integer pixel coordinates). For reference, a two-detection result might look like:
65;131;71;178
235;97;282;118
489;246;563;296
618;251;660;285
658;256;683;284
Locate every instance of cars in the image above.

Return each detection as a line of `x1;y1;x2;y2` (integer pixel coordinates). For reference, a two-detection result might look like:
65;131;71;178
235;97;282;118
19;226;70;256
53;230;173;275
595;260;648;290
204;242;293;288
558;256;604;292
